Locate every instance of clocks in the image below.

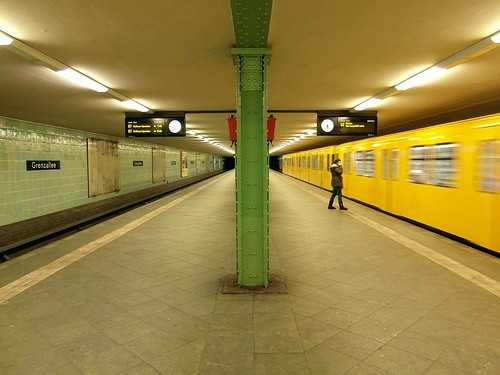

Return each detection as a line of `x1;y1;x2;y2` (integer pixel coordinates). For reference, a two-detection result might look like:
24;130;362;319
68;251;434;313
321;119;334;132
169;120;182;133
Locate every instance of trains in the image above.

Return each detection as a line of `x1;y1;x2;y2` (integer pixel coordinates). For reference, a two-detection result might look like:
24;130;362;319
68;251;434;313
279;113;500;256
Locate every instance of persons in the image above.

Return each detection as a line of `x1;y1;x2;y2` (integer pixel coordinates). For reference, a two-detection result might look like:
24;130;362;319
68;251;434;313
328;159;348;210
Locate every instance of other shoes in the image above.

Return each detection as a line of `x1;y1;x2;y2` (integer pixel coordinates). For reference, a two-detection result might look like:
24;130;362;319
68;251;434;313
340;206;348;210
328;205;336;209
